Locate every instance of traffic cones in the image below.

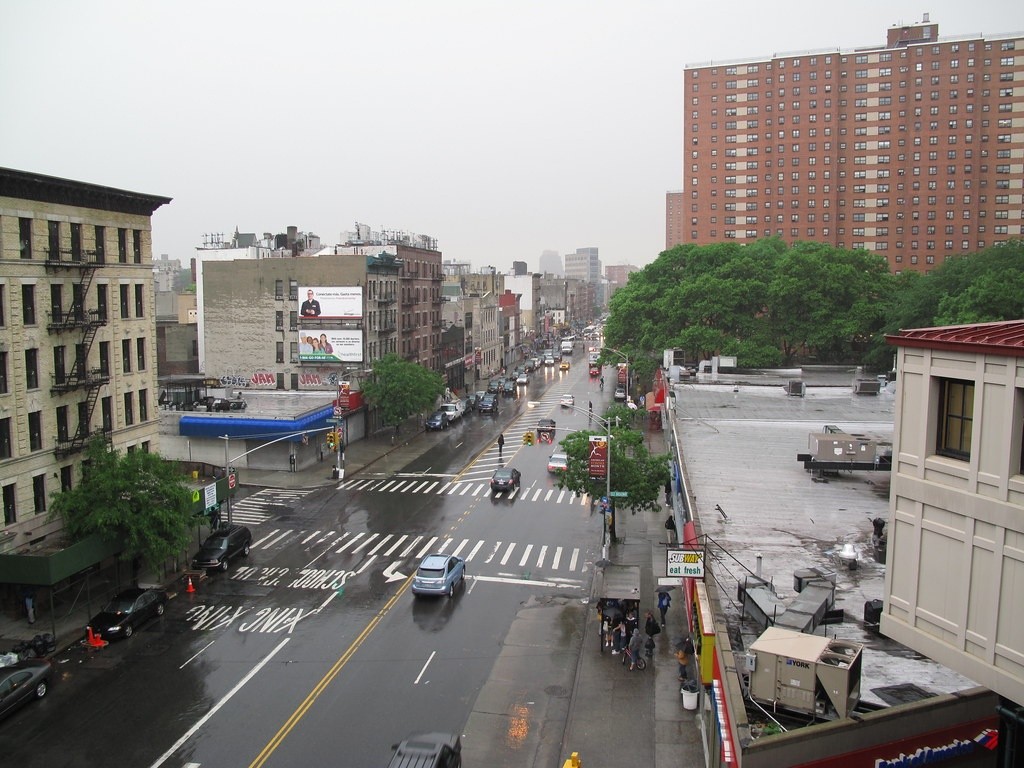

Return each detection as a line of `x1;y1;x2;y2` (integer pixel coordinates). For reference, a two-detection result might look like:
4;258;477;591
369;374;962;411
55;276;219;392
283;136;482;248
186;577;196;592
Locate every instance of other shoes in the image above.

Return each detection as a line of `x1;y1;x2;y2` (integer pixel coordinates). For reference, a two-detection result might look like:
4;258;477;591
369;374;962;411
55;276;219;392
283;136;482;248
662;624;664;629
612;650;620;654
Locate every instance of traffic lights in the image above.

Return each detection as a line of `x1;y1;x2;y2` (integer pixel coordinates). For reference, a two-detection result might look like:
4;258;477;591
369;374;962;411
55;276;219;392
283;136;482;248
326;432;339;447
523;431;534;446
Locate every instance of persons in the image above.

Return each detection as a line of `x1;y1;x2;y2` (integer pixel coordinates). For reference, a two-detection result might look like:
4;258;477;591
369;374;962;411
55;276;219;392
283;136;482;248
629;628;643;671
657;592;671;627
500;367;506;376
635;373;640;383
300;332;333;354
596;597;637;655
664;515;676;547
645;612;661;656
497;434;505;452
301;290;321;317
674;650;688;680
589;401;593;415
600;376;604;388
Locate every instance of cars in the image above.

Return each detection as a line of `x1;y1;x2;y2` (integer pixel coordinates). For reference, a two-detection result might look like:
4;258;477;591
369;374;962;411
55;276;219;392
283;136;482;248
86;586;166;637
411;553;465;598
491;467;521;492
426;411;449;431
388;729;462;768
560;394;575;406
547;454;569;473
0;658;55;722
614;388;626;399
537;419;557;432
191;524;253;573
441;317;607;424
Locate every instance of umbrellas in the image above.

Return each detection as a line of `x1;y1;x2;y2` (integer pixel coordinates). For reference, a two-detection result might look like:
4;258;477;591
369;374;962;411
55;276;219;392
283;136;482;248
595;560;617;568
603;606;624;619
655;585;676;592
672;633;695;655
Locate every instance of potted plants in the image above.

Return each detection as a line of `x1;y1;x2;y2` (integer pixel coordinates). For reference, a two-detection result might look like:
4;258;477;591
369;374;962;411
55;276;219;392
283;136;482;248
680;678;700;710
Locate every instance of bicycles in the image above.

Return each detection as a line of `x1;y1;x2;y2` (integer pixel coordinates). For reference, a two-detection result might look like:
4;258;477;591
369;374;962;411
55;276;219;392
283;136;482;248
621;645;647;671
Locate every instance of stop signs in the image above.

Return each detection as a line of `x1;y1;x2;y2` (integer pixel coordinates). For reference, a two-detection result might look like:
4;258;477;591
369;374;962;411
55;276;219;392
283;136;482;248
229;474;235;489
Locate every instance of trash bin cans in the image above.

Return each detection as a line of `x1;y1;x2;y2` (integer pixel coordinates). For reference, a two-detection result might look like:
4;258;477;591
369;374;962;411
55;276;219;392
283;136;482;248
681;689;699;710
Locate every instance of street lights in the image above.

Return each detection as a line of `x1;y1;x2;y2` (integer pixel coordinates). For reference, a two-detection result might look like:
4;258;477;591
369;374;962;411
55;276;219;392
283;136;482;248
588;347;628;402
334;368;373;468
528;401;610;504
